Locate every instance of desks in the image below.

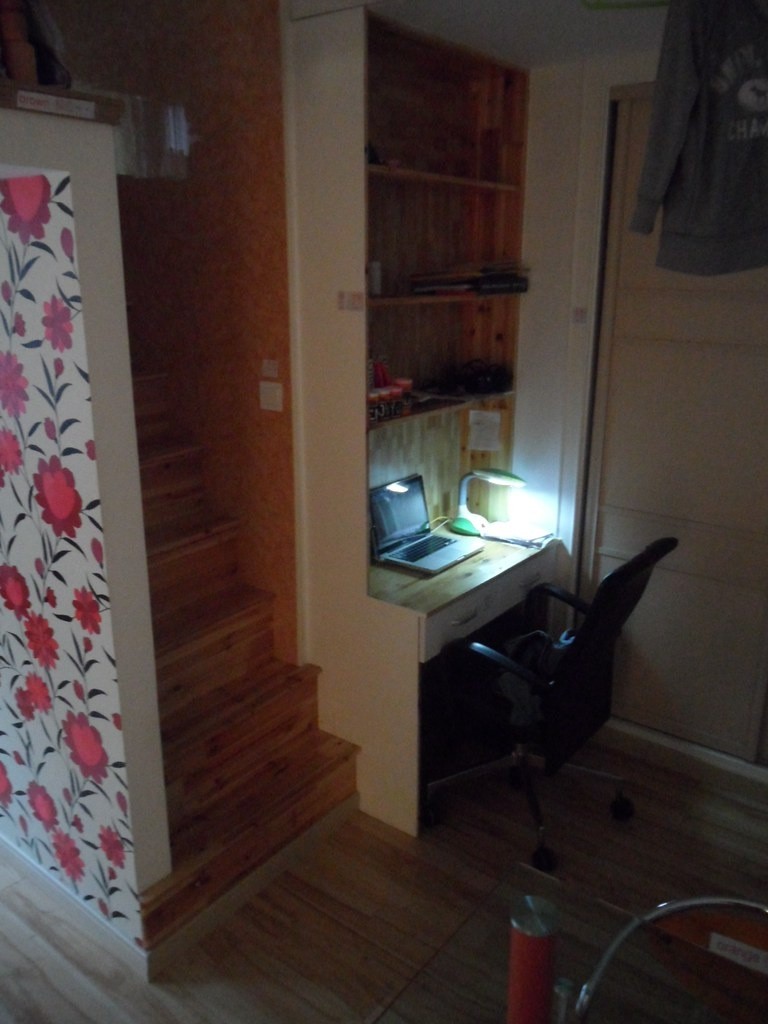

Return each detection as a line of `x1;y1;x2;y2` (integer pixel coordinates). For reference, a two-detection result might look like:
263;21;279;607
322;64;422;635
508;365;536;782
357;521;558;835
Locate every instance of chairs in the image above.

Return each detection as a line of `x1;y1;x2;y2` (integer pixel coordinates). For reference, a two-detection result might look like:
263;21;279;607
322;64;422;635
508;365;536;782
420;531;678;876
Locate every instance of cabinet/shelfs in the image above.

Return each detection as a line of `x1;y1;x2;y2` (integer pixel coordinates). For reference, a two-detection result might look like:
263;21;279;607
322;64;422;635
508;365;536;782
364;9;528;516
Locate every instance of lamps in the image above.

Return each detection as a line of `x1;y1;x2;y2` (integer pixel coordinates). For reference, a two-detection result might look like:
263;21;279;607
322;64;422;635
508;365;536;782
447;466;526;538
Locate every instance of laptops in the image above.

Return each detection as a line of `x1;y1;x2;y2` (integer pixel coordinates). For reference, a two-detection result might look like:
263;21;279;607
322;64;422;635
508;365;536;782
368;473;484;575
